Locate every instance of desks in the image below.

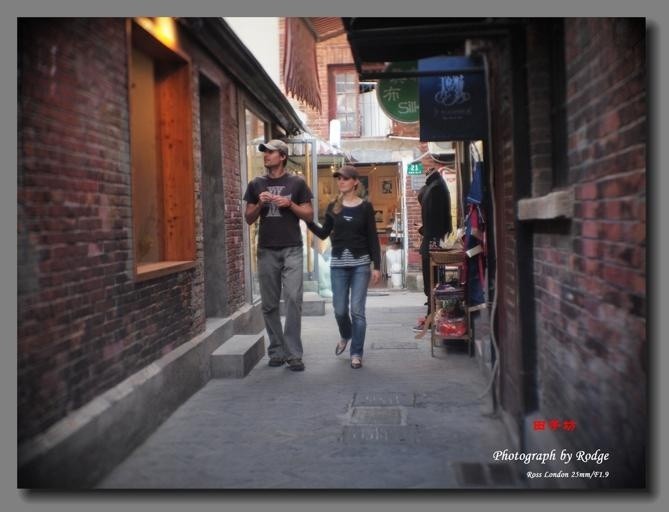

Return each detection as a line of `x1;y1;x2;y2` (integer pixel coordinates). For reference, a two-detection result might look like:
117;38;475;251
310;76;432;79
430;248;472;356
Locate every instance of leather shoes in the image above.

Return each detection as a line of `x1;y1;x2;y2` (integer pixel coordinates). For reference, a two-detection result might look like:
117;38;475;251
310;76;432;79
334;336;351;356
350;356;363;369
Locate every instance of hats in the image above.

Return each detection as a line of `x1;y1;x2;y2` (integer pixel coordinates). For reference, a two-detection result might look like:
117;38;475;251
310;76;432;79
258;138;290;155
332;165;359;182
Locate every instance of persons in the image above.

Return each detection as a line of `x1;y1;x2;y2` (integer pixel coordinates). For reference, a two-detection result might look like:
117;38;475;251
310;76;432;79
412;185;432;331
242;140;314;371
420;167;452;297
299;165;381;369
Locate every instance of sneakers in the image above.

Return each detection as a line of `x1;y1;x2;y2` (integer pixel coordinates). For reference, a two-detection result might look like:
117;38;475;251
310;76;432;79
268;354;286;367
287;356;305;372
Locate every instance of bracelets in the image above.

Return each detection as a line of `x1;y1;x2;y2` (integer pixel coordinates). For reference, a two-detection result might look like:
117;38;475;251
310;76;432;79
288;200;292;208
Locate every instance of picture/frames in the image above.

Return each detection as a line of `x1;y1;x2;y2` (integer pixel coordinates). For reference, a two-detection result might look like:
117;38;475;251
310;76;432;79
374;205;388;229
378;176;396;198
319;177;334;196
